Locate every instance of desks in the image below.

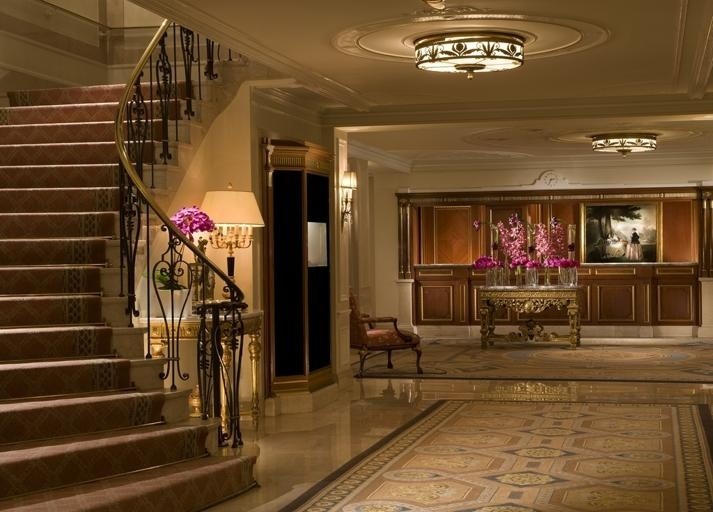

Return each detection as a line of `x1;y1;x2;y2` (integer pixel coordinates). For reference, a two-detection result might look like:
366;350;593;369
140;311;265;439
478;284;581;349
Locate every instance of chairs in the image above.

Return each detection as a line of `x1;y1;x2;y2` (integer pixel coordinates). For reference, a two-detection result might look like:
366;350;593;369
347;289;423;377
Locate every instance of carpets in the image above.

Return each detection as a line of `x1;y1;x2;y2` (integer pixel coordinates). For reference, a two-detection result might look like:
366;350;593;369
281;397;712;510
349;337;713;384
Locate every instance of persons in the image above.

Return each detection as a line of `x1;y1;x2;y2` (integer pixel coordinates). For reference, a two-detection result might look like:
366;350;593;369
611;232;627;241
627;228;642;262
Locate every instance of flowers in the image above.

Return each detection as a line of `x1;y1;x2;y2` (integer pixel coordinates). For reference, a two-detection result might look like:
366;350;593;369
167;206;217;247
470;211;580;270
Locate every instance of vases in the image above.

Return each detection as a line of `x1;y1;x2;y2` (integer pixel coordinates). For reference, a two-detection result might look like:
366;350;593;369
485;267;579;287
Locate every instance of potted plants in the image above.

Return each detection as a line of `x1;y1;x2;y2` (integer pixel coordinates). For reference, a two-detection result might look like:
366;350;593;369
142;265;192;320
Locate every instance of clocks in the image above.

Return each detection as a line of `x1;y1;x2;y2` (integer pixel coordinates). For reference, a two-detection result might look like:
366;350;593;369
541;171;560;189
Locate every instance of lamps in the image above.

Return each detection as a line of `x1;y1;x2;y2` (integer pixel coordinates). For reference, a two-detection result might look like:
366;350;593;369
200;182;265;312
339;169;358;231
590;133;658;159
409;31;528;81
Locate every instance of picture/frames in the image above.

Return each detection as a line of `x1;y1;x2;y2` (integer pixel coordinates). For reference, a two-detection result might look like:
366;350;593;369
578;201;664;263
